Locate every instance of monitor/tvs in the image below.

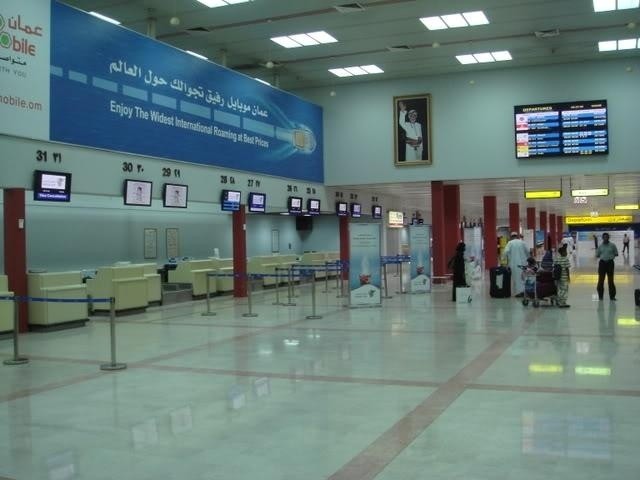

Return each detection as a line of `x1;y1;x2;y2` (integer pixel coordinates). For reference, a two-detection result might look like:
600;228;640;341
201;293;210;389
249;192;266;212
163;183;188;208
125;179;152;205
222;190;241;211
372;206;381;219
336;202;349;216
288;196;302;213
351;204;361;217
35;169;72;201
308;199;320;214
514;99;609;158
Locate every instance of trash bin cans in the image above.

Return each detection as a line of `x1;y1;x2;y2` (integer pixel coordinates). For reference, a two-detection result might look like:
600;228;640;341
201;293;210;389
456;286;472;304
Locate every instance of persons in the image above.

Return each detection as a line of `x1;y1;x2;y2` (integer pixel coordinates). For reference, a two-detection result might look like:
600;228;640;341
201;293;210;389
621;233;630;253
540;249;554;272
521;257;538;300
500;231;530;297
559;235;577;259
595;232;618;301
593;235;598;248
553;246;572;307
448;242;467;301
398;100;425;162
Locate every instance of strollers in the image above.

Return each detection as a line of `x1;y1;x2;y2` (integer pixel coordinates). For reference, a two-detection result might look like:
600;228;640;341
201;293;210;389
517;261;561;308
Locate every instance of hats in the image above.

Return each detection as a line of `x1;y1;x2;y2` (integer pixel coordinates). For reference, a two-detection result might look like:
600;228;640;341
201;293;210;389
510;231;517;236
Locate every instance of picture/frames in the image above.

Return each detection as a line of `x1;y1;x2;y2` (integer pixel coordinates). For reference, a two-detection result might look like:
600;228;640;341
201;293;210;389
392;94;433;168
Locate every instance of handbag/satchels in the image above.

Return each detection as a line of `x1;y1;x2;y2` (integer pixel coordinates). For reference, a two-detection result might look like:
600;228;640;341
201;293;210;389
447;258;455;268
553;263;562;280
455;286;471;303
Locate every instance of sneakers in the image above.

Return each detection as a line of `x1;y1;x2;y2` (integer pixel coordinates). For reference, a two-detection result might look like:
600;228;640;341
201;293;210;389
556;302;569;307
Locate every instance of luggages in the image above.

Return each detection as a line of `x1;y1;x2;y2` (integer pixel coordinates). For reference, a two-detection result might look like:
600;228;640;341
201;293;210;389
490;260;511;297
